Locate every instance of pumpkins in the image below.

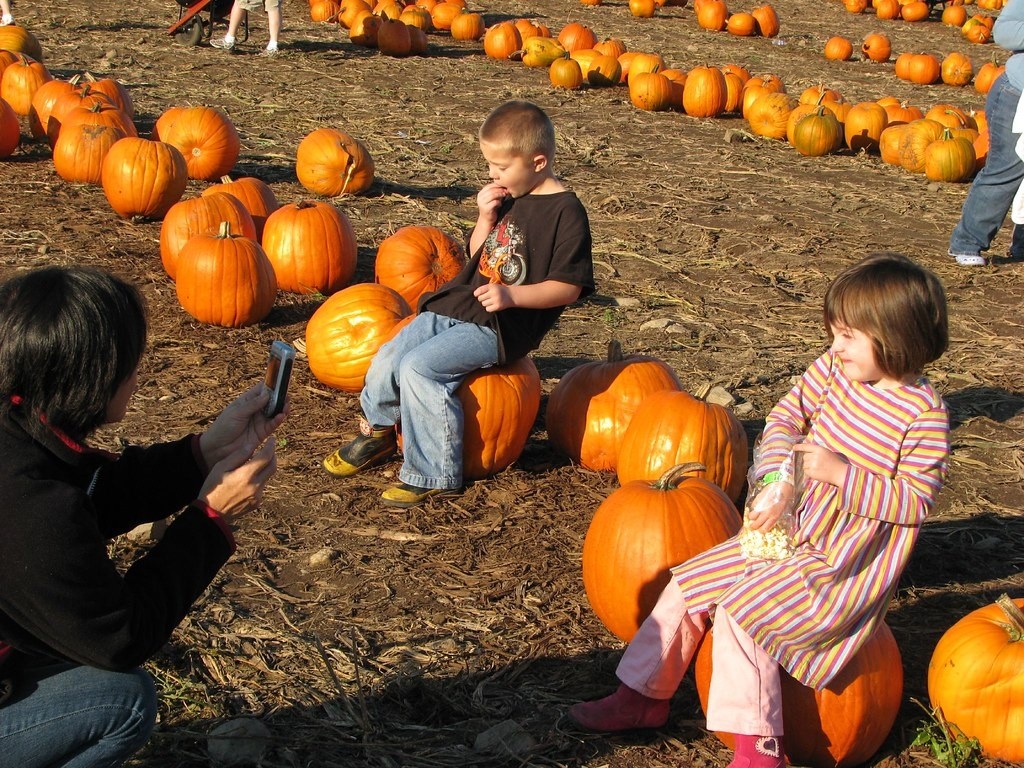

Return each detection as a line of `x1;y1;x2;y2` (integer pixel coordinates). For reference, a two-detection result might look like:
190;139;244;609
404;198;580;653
696;621;902;768
306;224;540;474
308;0;1006;182
546;342;748;645
0;24;374;328
928;593;1024;763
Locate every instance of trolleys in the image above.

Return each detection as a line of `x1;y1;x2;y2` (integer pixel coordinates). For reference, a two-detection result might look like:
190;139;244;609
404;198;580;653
168;0;248;47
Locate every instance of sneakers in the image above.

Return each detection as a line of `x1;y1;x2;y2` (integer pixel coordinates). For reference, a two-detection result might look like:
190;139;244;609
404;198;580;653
261;48;278;56
323;426;398;478
381;483;458;507
210;38;234;49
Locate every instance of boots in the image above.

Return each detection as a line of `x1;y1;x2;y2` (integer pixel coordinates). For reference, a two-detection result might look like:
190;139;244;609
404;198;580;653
569;683;668;731
724;734;786;768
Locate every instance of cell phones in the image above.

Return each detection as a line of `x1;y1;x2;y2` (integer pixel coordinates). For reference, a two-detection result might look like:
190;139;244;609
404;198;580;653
260;341;295;419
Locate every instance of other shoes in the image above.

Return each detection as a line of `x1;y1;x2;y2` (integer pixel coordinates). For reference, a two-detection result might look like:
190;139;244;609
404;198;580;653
948;248;985;266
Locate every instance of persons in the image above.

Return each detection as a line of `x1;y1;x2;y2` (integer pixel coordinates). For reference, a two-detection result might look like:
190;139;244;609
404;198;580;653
945;2;1024;268
324;100;591;508
567;249;951;768
0;0;22;27
0;262;294;768
208;0;283;57
